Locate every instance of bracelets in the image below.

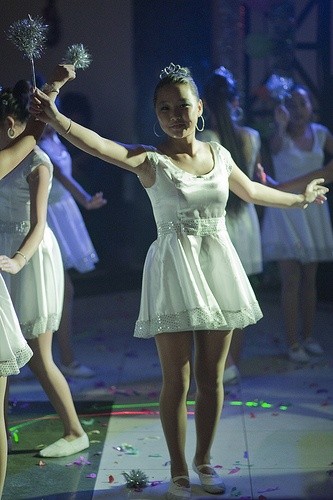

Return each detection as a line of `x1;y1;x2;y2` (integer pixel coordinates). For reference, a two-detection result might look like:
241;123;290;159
14;249;29;265
58;118;73;137
302;193;311;209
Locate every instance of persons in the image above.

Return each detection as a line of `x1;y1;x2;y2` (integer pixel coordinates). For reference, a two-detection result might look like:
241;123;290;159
0;270;34;498
36;124;108;380
260;84;332;364
24;64;330;500
0;76;90;459
195;66;263;379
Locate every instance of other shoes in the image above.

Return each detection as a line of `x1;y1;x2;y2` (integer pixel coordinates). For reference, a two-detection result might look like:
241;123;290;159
166;475;192;500
304;340;322;354
39;431;89;457
192;461;225;494
284;339;308;363
60;360;94;379
222;366;238;386
